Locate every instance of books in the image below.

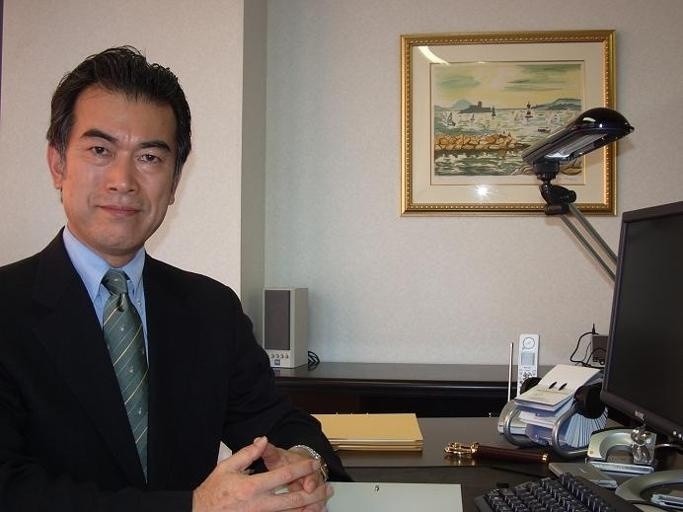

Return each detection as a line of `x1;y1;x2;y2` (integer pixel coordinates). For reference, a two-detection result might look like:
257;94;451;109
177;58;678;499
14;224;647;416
309;411;424;452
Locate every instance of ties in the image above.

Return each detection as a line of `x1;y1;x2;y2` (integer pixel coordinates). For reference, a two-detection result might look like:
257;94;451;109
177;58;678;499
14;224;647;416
98;270;149;487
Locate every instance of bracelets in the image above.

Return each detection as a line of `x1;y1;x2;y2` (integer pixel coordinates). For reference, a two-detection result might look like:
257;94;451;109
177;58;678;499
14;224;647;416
296;446;330;483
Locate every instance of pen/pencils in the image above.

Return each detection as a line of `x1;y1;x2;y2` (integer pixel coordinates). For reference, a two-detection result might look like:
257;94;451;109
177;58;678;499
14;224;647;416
443;441;551;464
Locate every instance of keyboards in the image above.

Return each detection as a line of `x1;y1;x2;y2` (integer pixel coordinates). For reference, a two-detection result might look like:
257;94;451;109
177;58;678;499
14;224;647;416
471;471;646;512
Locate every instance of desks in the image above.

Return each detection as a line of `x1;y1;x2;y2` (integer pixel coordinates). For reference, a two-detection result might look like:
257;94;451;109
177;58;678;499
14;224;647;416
313;413;681;510
271;360;557;407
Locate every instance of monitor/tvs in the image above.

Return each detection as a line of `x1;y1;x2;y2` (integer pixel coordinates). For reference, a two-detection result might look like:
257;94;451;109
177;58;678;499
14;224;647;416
599;201;683;512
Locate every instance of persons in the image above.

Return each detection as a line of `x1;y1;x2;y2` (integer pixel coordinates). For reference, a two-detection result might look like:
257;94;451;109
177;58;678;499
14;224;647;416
0;43;353;511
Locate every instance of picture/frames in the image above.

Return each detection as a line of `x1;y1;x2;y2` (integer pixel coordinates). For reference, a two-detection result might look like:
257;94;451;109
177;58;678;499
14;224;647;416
399;28;618;219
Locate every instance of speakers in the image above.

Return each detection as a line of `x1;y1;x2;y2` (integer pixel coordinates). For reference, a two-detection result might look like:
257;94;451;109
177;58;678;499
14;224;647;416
260;286;310;370
